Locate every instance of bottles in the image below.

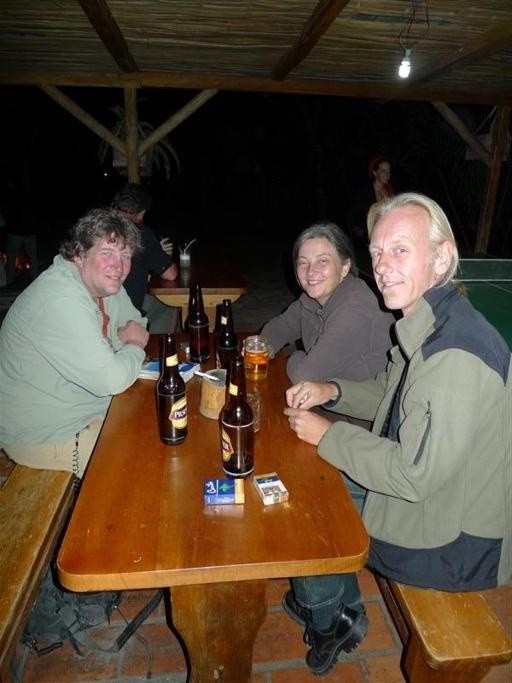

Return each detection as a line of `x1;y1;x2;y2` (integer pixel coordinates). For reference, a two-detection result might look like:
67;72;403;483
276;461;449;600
155;332;190;446
186;278;212;364
214;298;240;372
219;351;258;478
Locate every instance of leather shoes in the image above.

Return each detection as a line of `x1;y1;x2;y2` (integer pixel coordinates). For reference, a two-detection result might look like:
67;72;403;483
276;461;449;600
282;589;366;626
299;604;369;676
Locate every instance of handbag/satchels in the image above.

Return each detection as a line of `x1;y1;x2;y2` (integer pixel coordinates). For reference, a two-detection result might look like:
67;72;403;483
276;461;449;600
24;553;120;642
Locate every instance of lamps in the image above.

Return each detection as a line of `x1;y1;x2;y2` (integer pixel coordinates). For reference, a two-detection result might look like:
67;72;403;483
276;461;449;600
394;0;429;81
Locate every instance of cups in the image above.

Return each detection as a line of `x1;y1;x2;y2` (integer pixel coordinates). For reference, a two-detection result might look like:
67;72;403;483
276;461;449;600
178;246;192;268
160;232;174;256
244;332;269;382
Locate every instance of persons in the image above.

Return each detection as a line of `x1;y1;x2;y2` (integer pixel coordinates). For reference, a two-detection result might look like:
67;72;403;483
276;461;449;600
241;221;403;430
110;191;183;334
367;152;398;204
1;205;152;481
282;191;512;678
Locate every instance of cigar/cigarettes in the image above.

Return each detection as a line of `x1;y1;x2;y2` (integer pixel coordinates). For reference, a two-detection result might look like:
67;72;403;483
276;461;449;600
194;371;220;381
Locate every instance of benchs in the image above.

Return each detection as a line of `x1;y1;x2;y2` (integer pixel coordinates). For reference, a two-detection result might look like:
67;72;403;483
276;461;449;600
0;448;78;683
368;559;512;683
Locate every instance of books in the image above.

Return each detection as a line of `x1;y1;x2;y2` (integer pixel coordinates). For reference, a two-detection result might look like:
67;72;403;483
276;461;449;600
138;359;200;384
